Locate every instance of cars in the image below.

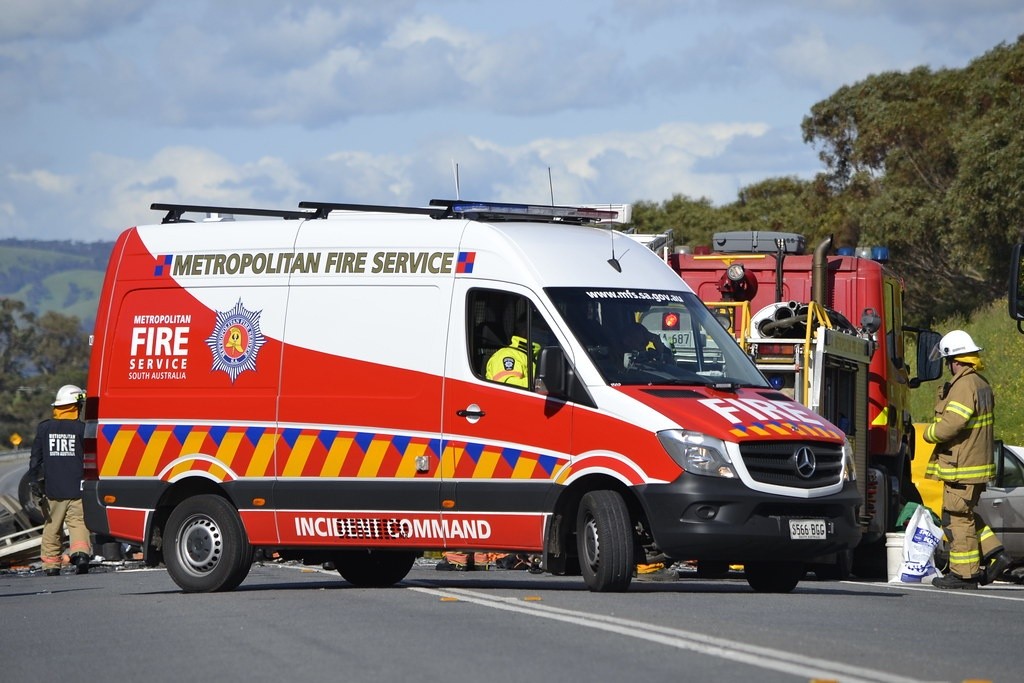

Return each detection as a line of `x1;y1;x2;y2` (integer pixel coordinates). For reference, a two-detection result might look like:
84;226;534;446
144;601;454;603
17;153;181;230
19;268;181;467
973;446;1024;560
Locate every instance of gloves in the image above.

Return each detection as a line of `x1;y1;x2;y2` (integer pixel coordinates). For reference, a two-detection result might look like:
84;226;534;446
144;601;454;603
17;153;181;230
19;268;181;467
29;481;43;498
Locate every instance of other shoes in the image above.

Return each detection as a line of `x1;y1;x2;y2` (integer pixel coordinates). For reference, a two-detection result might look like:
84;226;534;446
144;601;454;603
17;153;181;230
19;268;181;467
76;557;88;574
435;563;476;572
529;556;543;575
496;555;529;570
46;569;60;576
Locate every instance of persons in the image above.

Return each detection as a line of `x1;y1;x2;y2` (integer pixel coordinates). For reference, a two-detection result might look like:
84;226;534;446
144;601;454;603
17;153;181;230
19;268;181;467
922;330;1011;591
30;384;95;576
485;295;566;394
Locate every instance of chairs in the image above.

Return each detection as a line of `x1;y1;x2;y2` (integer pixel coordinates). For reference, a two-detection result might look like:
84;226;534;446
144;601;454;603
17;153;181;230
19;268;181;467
474;320;509;378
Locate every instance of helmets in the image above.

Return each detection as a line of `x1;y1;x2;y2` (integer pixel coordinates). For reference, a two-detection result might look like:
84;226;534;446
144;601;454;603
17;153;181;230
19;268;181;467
939;330;982;358
51;384;86;406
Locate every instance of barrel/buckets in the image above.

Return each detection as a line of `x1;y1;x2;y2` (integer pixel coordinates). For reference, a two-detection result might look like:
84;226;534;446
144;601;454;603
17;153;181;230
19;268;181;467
886;533;905;583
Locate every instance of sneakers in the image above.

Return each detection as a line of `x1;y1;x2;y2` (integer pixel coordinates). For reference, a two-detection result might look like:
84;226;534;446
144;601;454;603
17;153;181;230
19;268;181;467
978;549;1014;585
932;572;978;589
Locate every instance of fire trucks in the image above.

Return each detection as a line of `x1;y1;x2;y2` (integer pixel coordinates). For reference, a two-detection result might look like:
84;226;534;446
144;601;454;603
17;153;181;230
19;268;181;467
678;229;949;573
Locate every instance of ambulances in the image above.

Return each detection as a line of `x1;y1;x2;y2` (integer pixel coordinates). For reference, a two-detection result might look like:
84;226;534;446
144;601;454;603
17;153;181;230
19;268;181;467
77;199;868;596
